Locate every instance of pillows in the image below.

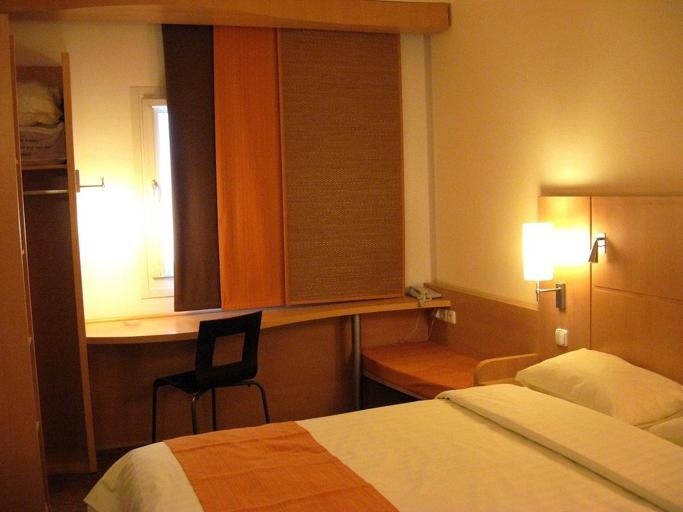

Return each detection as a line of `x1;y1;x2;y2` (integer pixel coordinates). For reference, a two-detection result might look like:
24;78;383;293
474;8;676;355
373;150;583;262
515;348;682;427
647;416;683;446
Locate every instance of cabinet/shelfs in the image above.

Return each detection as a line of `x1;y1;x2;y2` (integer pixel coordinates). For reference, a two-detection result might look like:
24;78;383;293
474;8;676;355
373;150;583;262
0;12;97;512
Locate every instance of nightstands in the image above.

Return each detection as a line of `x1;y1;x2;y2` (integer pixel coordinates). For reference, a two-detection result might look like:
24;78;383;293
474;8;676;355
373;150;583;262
351;341;536;413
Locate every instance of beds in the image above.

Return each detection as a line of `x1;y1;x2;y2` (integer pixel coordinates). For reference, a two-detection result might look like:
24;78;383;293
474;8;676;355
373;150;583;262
82;192;683;511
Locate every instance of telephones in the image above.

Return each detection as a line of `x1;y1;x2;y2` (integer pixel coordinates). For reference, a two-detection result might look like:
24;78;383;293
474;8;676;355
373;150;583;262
408;284;442;307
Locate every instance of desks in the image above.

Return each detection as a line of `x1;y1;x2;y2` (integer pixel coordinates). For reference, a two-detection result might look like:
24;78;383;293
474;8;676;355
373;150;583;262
84;295;451;451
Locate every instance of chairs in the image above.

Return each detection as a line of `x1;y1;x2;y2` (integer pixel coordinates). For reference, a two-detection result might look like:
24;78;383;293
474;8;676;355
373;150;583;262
151;308;269;444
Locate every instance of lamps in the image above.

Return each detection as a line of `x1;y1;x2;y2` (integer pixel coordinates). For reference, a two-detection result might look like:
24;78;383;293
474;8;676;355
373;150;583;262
588;232;607;264
522;220;566;311
